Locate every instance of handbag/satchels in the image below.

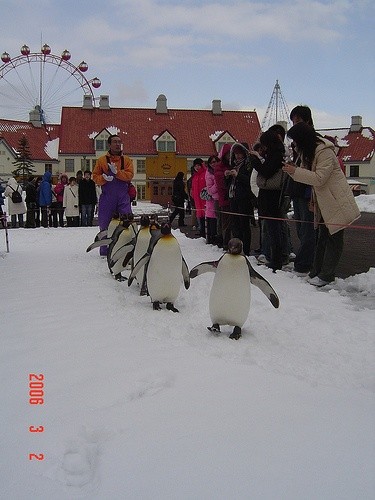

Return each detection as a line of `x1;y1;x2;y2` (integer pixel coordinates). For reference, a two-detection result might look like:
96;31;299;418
172;194;179;203
200;188;211;200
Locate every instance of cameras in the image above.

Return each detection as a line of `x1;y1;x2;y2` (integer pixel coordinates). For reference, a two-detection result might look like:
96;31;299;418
228;171;233;175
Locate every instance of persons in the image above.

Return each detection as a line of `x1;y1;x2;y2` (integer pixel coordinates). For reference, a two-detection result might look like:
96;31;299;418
92;134;134;258
323;136;345;172
0;184;11;227
185;125;291;269
171;172;189;226
25;170;96;228
286;105;325;274
3;177;26;229
282;122;361;286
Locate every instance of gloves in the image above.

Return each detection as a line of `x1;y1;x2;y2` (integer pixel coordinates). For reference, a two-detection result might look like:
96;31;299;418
102;173;113;181
108;162;118;174
63;207;66;209
74;205;77;208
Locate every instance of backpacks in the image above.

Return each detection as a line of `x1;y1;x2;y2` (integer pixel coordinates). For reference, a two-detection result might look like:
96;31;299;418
8;185;22;203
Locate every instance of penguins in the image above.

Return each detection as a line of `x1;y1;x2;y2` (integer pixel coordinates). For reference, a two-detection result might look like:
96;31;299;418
85;208;192;314
189;237;280;342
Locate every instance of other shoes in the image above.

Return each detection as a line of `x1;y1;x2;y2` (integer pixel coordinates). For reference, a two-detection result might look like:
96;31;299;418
289;252;296;259
178;224;188;227
307;276;328;287
